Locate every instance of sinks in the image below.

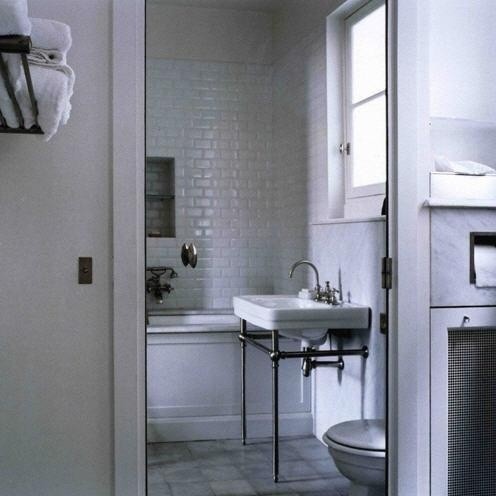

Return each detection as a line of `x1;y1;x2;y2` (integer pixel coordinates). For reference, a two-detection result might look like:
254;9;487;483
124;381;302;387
232;294;369;331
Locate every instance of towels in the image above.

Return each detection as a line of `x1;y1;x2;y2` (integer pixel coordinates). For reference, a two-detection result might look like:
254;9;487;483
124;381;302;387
0;1;75;143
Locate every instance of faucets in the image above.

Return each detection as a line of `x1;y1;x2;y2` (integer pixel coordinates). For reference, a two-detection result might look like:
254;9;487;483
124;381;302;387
288;259;320;302
154;289;164;304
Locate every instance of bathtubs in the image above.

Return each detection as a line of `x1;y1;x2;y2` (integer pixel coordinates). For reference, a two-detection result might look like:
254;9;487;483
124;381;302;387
147;308;313;443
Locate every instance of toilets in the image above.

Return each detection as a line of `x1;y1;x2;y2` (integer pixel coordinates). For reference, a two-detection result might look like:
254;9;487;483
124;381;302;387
322;419;386;495
